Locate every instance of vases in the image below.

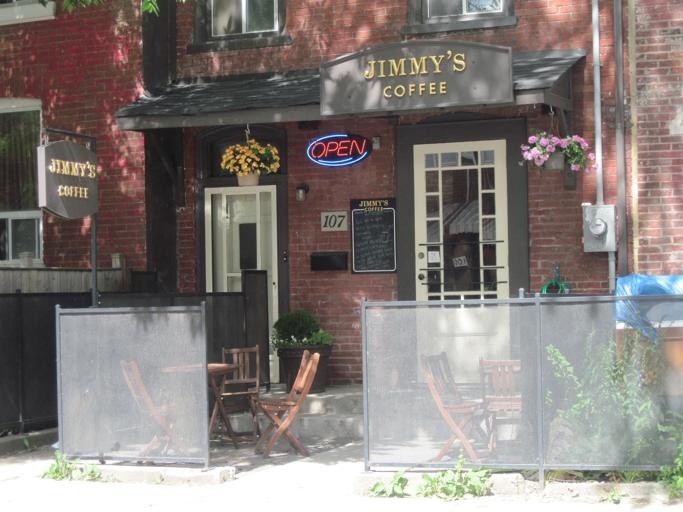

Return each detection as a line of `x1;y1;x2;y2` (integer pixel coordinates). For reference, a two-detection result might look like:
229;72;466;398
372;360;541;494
276;344;333;394
543;149;565;171
237;169;261;187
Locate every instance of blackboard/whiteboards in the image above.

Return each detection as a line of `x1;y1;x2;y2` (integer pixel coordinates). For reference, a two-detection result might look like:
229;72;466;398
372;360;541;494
348;199;397;274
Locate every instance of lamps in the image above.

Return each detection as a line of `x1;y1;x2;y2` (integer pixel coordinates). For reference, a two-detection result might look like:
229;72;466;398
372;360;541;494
296;183;309;202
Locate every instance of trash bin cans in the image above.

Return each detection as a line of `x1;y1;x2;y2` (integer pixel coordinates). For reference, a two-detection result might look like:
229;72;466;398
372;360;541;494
616;328;682;414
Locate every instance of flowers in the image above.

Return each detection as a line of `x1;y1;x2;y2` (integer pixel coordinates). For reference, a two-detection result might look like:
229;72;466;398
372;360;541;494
221;139;280;176
268;313;333;349
519;130;596;176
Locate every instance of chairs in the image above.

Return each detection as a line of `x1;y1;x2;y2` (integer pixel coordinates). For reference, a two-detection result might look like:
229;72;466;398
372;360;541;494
478;357;524;460
218;344;262;444
253;350;320;457
420;351;494;463
173;345;223;443
119;360;203;462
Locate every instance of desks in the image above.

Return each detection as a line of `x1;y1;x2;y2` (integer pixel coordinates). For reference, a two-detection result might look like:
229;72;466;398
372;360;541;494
162;362;239;470
